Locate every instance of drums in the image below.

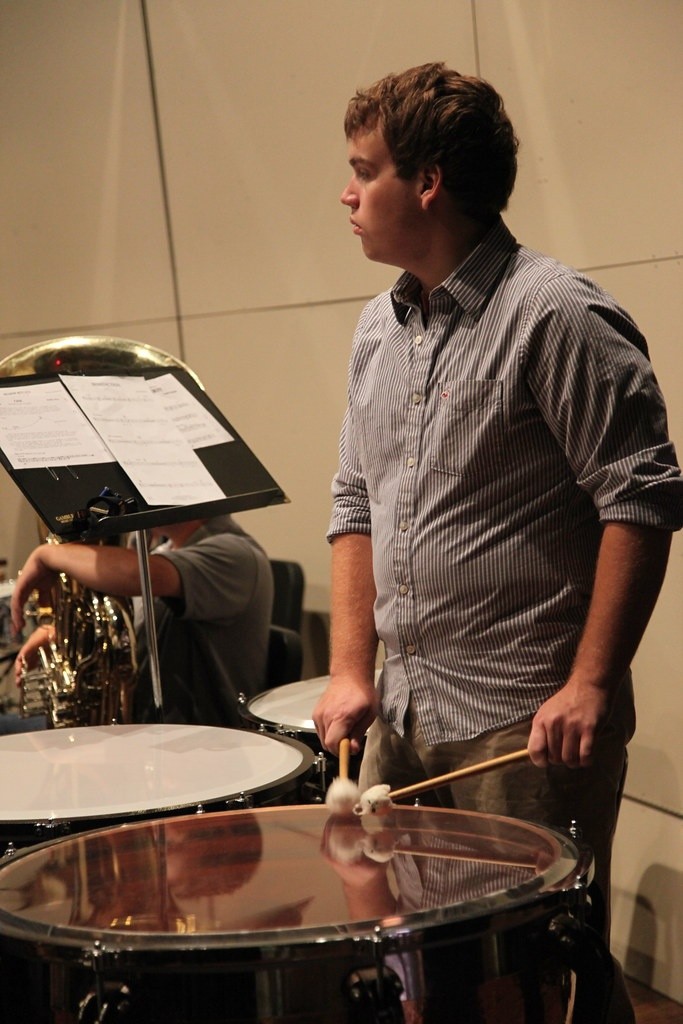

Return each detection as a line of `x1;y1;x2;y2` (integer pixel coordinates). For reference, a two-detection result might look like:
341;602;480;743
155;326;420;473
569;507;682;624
1;669;638;1024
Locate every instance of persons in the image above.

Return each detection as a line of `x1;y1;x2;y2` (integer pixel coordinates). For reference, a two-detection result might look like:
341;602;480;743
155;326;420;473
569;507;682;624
9;512;275;735
312;60;683;1023
318;806;552;919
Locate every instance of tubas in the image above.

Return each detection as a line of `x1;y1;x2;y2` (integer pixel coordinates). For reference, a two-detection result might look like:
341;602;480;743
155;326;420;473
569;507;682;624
0;333;207;730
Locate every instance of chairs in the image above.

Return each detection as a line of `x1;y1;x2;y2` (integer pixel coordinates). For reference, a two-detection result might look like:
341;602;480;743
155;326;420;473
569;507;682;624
262;560;308;692
